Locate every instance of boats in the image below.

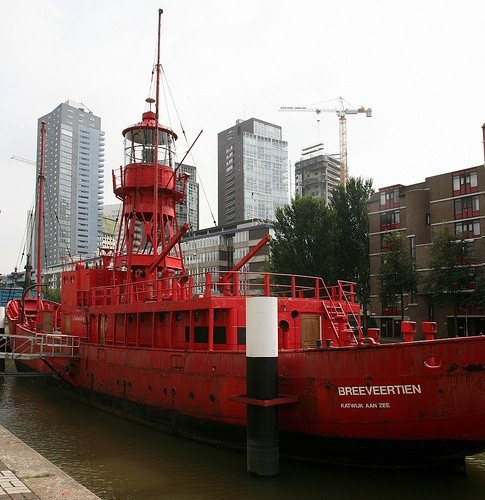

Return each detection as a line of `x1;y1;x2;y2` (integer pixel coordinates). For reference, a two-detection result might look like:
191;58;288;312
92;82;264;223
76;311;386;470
3;8;484;470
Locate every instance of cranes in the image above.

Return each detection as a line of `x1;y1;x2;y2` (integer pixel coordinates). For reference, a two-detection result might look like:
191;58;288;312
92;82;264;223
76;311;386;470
278;95;372;193
